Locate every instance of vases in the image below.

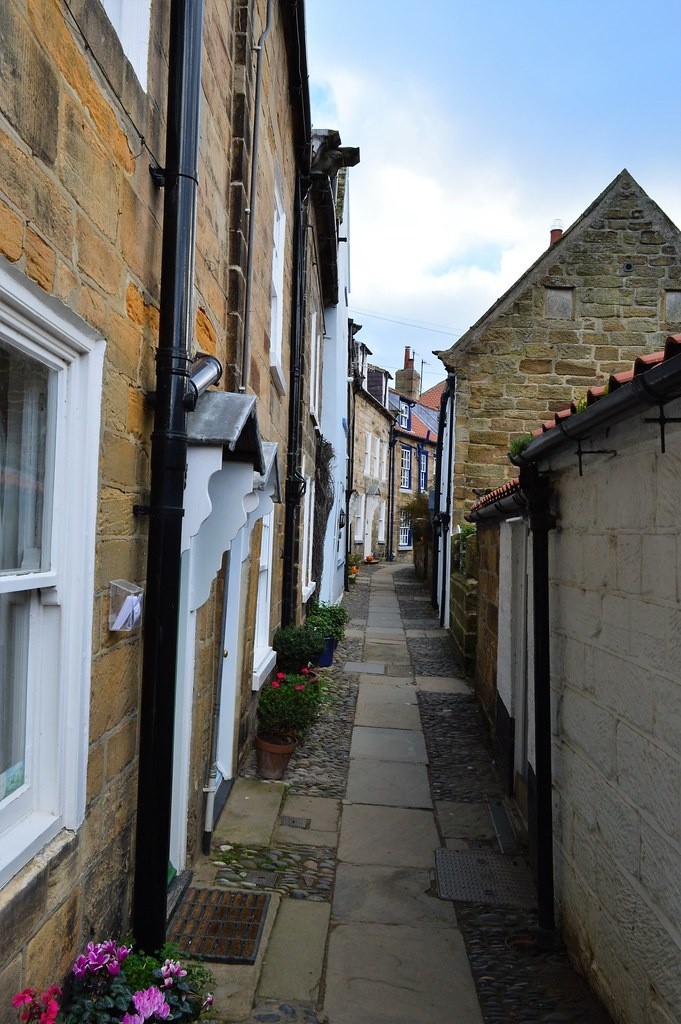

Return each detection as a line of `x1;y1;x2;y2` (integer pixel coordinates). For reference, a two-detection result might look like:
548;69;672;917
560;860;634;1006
307;671;319;692
254;730;298;780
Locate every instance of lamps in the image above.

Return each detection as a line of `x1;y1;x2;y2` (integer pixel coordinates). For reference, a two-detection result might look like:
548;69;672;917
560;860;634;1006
188;350;223;412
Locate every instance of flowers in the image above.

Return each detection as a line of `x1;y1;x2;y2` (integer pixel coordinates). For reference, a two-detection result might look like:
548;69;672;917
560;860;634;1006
255;669;320;744
65;930;145;997
11;983;82;1024
348;566;357;575
131;941;220;1018
82;985;174;1024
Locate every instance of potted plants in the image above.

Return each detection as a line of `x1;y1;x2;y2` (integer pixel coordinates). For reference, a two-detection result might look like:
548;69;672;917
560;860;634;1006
305;614;346;667
273;622;328;673
